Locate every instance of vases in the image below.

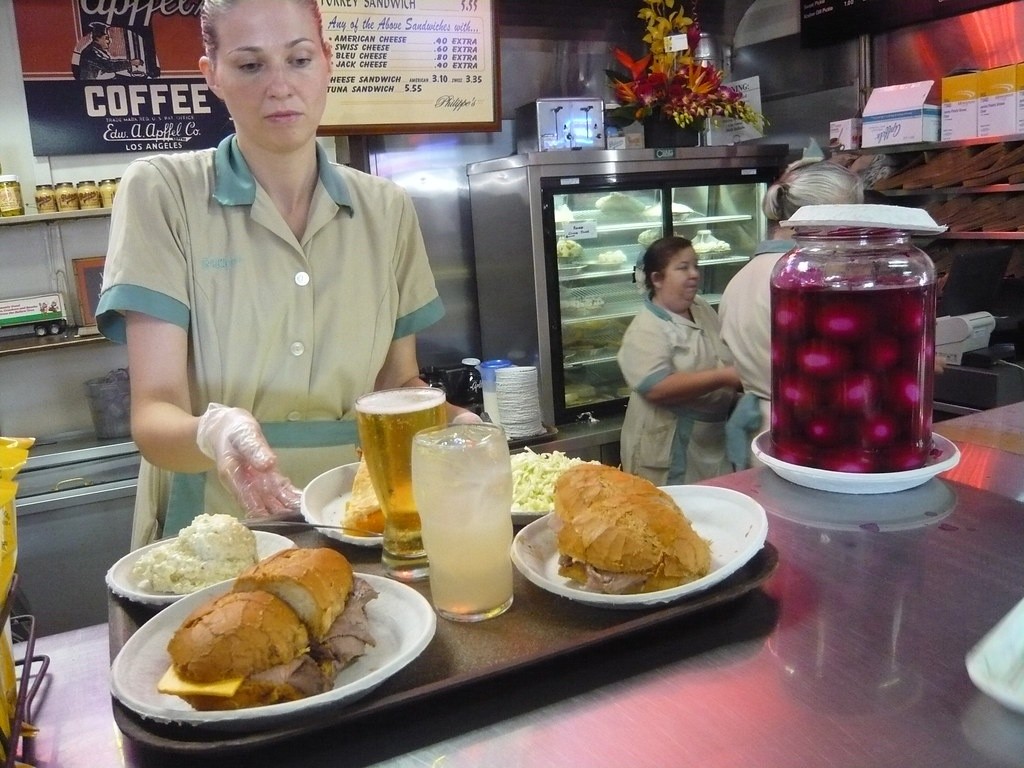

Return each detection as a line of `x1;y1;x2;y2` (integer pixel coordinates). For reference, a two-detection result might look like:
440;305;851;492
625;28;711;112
644;105;699;148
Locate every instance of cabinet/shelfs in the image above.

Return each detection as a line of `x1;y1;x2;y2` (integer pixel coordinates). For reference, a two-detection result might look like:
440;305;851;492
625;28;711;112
831;132;1024;419
467;143;792;429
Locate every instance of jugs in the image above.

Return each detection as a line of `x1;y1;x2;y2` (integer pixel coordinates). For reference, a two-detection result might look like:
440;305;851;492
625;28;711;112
476;358;512;423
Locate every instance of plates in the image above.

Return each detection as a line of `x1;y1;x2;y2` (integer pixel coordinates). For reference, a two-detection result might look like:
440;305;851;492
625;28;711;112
759;468;958;530
110;572;435;727
495;367;542;436
508;509;551;525
106;529;298;604
779;205;948;232
555;203;648;276
301;462;383;548
511;485;769;610
751;427;960;493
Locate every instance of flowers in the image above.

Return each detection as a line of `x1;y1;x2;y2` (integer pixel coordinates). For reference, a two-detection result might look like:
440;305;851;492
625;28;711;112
599;0;772;131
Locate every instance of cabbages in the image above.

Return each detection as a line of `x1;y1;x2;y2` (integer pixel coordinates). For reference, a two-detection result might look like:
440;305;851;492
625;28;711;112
510;446;602;513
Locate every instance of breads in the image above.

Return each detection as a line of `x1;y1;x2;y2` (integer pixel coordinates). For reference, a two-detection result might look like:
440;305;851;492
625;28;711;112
340;450;386;537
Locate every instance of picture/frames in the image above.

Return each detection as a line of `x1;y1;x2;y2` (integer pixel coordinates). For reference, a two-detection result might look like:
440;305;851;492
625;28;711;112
72;255;107;327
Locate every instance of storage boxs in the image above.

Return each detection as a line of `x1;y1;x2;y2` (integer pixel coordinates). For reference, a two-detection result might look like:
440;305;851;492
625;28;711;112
830;60;1024;152
699;76;763;147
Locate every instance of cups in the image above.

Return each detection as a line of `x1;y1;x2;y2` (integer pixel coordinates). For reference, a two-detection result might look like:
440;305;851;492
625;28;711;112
355;386;447;579
410;426;514;621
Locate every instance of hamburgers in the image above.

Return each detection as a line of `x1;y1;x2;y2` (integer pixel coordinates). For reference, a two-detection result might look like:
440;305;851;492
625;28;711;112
553;465;712;593
158;546;380;712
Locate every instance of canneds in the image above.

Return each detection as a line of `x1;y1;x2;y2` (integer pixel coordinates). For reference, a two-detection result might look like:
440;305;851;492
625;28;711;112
35;177;122;213
0;174;25;217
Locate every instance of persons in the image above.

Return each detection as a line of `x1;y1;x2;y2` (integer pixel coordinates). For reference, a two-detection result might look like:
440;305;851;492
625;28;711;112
719;161;944;468
620;236;743;486
96;0;482;552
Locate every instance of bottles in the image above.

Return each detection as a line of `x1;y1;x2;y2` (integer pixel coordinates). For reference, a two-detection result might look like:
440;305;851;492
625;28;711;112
767;226;938;473
36;176;121;214
0;175;25;216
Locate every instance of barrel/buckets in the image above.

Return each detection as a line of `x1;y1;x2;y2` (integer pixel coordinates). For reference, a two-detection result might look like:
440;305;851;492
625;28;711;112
81;381;131;439
